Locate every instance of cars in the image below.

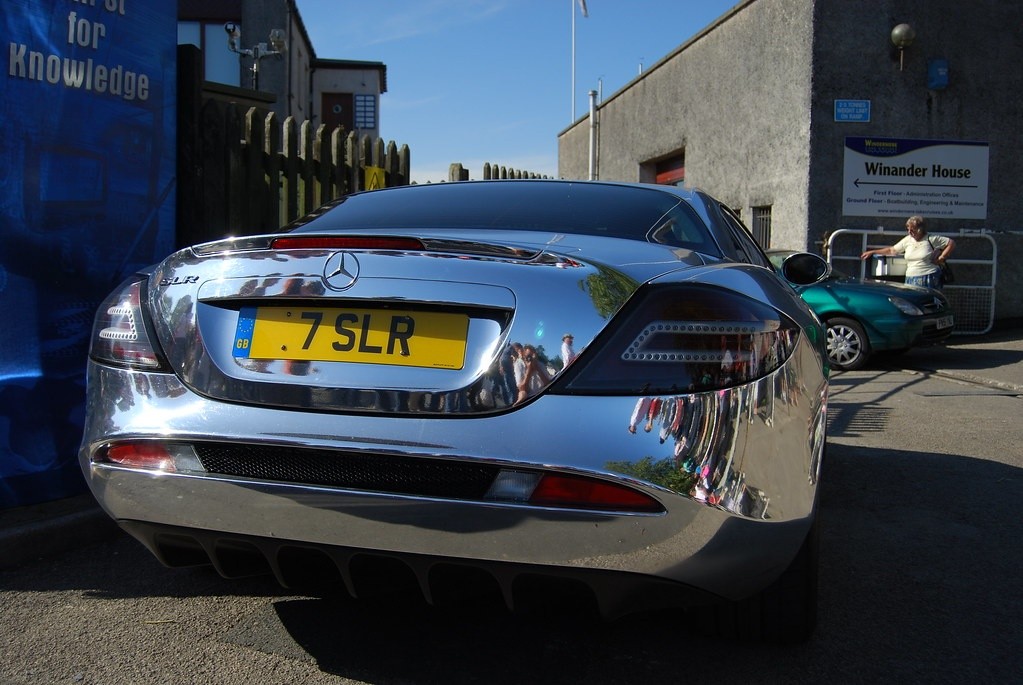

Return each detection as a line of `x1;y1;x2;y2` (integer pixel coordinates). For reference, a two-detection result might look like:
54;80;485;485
73;176;833;610
763;248;954;371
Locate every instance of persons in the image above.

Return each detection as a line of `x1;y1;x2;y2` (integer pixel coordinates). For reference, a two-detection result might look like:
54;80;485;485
239;273;324;297
152;295;210;392
861;215;956;293
505;333;575;409
375;349;504;412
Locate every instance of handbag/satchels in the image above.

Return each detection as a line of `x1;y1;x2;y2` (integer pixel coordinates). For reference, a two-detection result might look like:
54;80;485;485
928;234;954;284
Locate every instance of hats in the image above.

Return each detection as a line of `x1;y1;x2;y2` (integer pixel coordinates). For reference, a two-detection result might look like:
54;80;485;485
562;334;574;340
